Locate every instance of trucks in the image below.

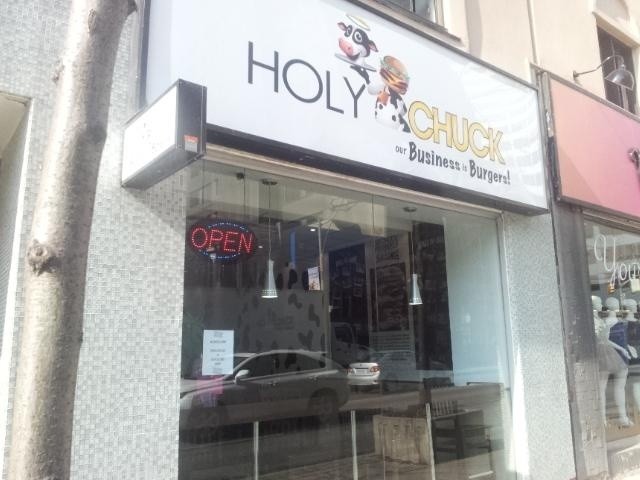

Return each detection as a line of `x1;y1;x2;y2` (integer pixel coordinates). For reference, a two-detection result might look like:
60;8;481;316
330;319;358;362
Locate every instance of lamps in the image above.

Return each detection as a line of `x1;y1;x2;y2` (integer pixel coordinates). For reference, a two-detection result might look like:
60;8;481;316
404;206;423;304
259;179;279;299
573;55;635;89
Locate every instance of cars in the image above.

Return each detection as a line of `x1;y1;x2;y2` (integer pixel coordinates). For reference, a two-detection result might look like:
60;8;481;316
348;346;452;393
171;349;351;439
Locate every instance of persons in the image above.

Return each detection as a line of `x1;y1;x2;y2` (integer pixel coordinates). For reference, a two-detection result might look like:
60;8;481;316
589;295;639;431
